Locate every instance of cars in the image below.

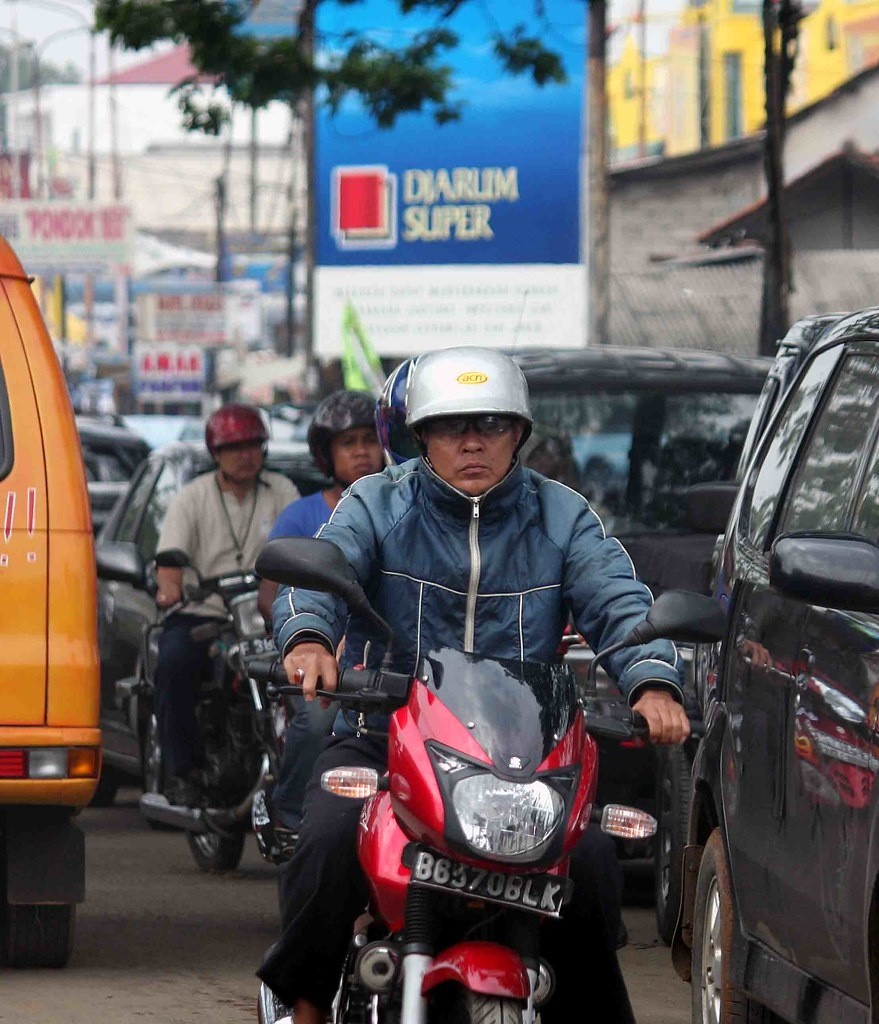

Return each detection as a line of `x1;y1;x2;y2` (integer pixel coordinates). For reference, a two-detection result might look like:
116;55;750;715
64;302;878;1021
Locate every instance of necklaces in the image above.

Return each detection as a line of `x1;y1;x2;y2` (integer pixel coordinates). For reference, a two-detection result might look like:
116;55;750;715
215;474;258;560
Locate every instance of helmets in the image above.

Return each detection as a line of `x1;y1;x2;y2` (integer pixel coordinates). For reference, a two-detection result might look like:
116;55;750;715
404;346;535;440
206;405;270;454
373;357;419;467
305;387;382;476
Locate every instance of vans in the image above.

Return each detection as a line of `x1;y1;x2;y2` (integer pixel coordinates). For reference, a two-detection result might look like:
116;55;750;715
2;239;102;971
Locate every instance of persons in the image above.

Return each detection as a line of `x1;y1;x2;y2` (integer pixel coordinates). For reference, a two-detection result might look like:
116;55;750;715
256;348;691;1024
155;404;302;808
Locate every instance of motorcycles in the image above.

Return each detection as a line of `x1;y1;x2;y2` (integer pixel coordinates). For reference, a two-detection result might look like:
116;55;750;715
139;554;363;873
252;537;733;1023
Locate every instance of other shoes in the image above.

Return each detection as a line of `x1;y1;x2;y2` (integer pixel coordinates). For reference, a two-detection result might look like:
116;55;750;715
178;770;212;801
267;818;299;862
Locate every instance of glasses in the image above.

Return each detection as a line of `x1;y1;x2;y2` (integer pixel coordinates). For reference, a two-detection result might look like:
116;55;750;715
425;415;516;438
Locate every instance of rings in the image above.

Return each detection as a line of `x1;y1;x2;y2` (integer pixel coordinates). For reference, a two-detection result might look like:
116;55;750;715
159;594;167;601
297;668;305;678
